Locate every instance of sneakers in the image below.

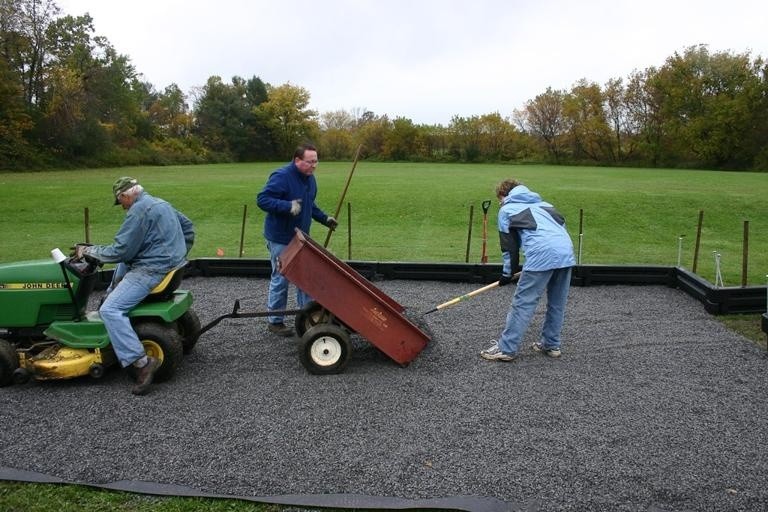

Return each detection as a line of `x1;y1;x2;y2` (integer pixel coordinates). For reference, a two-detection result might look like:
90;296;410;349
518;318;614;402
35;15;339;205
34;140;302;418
268;322;292;336
481;343;512;361
533;342;561;357
132;356;161;395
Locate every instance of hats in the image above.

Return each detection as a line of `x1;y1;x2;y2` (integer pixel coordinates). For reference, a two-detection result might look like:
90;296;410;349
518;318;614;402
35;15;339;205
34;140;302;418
112;176;138;205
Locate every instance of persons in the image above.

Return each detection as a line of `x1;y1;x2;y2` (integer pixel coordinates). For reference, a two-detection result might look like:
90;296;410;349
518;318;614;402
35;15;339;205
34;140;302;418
256;143;338;336
477;178;577;362
68;176;195;395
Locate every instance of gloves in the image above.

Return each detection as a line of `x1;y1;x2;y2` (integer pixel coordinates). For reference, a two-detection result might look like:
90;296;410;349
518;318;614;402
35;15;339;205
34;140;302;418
326;217;338;231
290;199;302;216
68;245;90;264
499;276;512;286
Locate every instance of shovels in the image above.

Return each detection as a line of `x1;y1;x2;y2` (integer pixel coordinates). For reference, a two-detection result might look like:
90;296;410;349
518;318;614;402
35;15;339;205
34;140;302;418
481;200;492;263
